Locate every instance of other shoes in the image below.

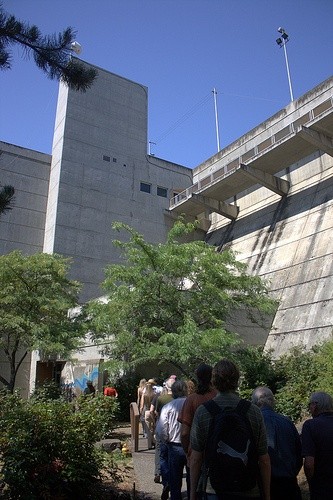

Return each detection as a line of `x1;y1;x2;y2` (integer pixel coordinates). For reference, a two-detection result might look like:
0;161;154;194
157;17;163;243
154;474;160;483
161;482;170;500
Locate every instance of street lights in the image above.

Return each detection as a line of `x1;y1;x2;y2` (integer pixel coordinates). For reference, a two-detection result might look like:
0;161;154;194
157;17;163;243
274;27;294;102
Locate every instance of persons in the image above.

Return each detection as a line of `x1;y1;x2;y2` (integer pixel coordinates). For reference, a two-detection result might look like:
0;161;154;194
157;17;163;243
83;381;118;400
188;360;272;499
303;391;333;500
248;387;304;500
137;374;197;500
179;364;218;500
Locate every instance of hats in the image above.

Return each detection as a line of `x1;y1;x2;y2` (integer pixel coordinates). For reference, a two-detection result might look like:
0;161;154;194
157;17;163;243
146;379;157;385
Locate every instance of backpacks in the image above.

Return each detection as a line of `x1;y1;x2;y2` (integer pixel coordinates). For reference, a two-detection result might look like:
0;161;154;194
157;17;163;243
202;399;259;493
